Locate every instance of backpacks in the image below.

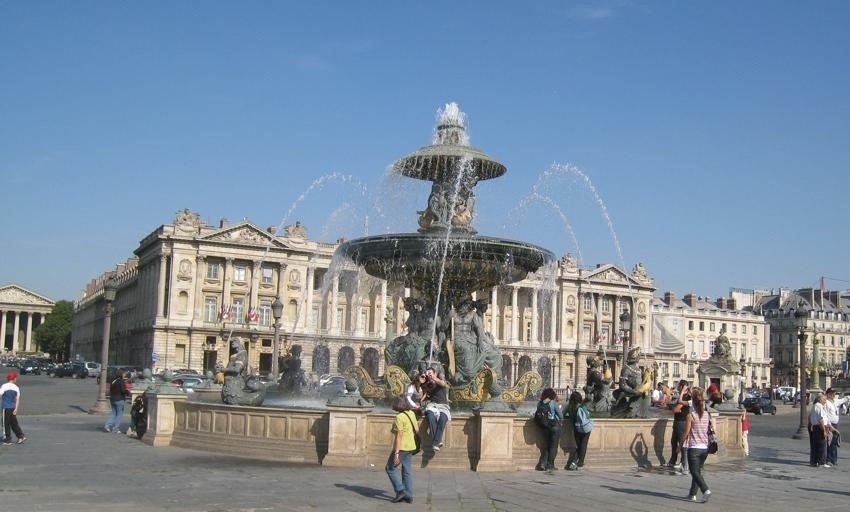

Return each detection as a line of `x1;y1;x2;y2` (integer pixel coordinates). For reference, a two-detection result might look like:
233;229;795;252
574;406;593;434
535;399;556;429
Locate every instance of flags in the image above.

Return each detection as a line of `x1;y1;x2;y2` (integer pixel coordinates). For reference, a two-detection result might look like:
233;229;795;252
220;304;233;321
247;306;260;322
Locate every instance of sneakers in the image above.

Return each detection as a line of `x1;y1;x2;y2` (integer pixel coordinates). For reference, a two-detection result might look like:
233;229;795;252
811;462;832;468
392;490;413;504
537;462;585;470
687;492;697;501
433;442;443;451
2;437;27;446
703;488;712;503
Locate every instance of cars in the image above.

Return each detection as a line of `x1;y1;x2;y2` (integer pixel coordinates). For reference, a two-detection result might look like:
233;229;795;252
0;354;143;383
144;363;385;393
740;385;799;416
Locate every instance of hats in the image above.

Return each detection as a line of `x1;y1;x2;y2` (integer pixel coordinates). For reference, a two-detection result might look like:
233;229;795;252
825;388;836;394
6;372;17;380
708;384;718;391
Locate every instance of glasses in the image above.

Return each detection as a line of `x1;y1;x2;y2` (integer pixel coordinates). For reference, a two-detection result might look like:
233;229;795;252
420;374;427;380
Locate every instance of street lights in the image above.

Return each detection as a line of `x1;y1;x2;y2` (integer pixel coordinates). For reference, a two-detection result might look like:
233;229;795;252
270;294;284;386
651;360;659;389
792;295;812;440
619;308;633;370
511;347;519;385
551;355;557;390
739;354;746;402
86;274;119;415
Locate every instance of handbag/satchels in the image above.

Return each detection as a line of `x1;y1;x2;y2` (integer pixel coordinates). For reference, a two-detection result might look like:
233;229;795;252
706;412;718;454
411;432;421;455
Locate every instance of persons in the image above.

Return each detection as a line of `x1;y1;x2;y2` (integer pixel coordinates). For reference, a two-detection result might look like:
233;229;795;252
742;408;750;456
585;355;615;411
538;386;564;471
104;368;129;434
0;373;27;444
216;337;248;391
776;384;850;467
614;348;652;420
383;367;451;504
652;379;727;503
715;329;730;357
562;390;591;470
